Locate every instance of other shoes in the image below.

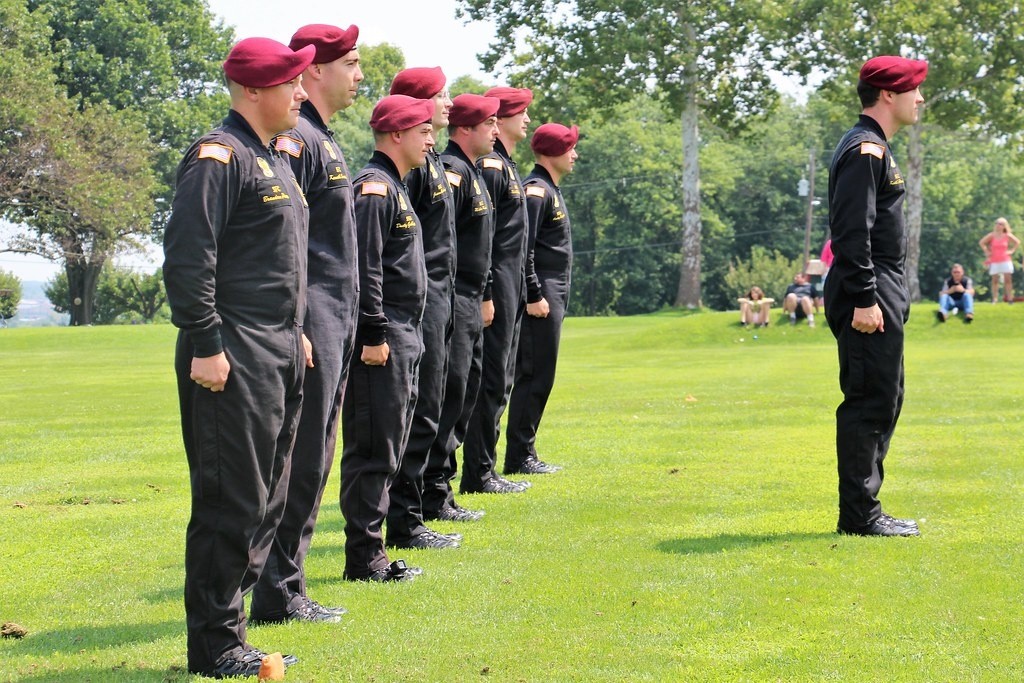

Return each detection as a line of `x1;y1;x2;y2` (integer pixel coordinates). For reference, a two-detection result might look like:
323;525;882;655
966;314;972;321
937;312;945;322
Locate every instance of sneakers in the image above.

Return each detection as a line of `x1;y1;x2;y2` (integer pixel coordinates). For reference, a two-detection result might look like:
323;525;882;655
204;647;298;678
274;600;347;624
406;528;462;549
368;563;423;583
482;475;531;493
519;459;560;473
861;514;919;537
438;505;485;522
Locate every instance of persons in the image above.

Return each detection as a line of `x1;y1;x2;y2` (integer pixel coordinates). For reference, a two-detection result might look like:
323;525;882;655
979;218;1020;305
738;287;774;327
938;263;975;323
785;273;819;327
163;24;578;679
826;55;929;536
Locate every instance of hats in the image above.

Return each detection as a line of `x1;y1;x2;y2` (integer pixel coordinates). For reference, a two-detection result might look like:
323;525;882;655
369;94;434;132
390;66;446;98
448;93;500;126
485;86;534;116
224;37;317;88
860;55;928;93
531;122;579;156
288;23;359;65
